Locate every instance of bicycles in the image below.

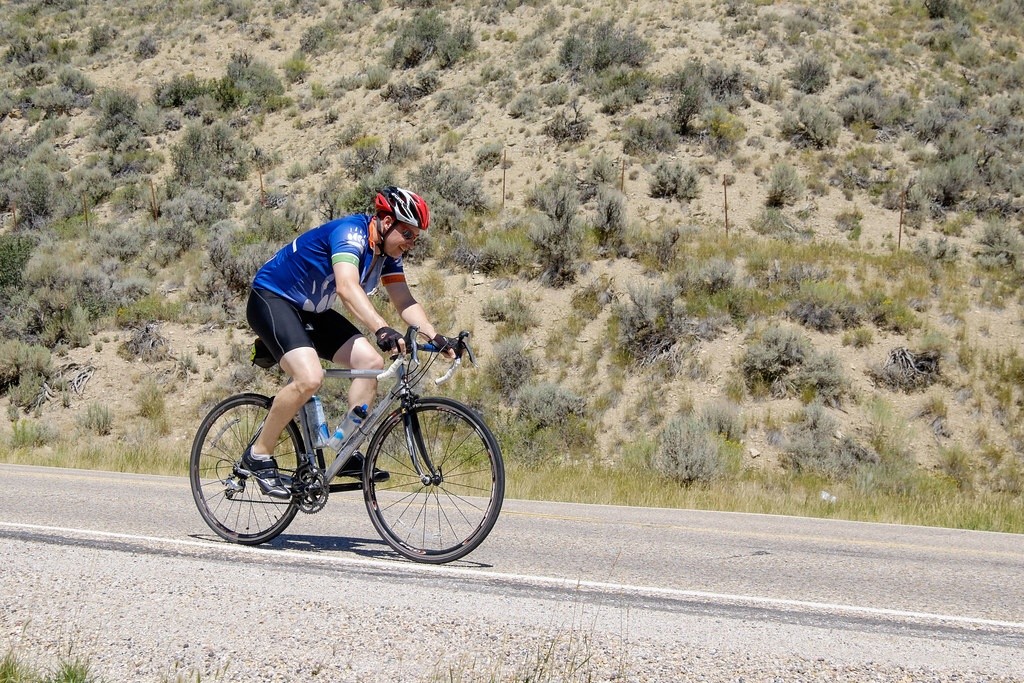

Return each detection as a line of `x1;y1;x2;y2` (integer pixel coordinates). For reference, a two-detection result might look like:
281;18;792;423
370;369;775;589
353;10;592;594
189;325;505;565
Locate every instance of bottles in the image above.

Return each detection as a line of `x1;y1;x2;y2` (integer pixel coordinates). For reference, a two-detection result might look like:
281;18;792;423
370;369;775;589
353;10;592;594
304;395;330;448
329;404;368;450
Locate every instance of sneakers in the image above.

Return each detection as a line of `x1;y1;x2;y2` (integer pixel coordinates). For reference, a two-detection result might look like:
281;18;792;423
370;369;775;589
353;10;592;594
240;446;291;499
335;451;390;482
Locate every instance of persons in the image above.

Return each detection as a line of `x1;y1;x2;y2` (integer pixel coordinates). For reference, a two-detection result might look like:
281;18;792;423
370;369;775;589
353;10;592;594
241;185;459;499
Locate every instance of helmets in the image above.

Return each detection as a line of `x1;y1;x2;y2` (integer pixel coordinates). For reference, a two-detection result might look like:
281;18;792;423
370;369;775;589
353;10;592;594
375;185;430;230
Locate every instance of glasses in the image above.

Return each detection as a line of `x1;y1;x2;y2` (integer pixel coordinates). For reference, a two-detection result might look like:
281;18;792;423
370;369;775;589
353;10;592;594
401;225;420;239
